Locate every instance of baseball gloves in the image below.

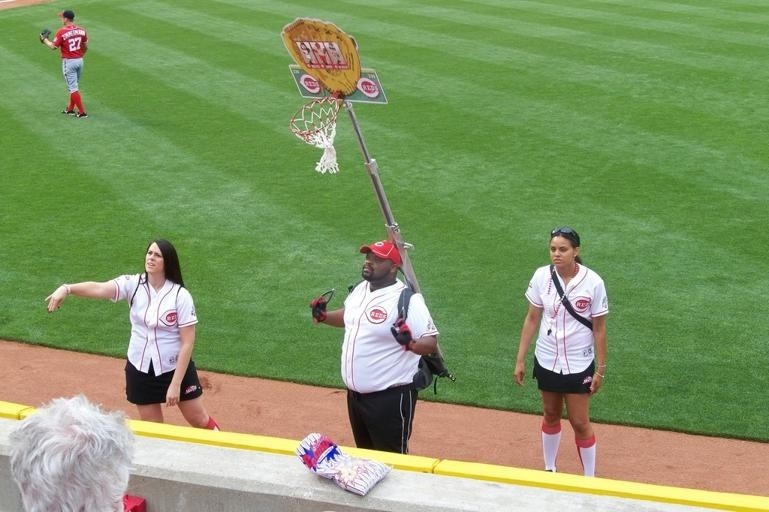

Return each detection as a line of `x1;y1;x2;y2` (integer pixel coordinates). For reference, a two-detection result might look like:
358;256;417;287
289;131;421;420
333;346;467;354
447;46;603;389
39;29;51;43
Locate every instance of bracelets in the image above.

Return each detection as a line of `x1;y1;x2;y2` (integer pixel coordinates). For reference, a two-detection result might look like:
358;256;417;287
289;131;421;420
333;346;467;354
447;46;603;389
63;282;71;295
597;365;606;367
595;371;604;378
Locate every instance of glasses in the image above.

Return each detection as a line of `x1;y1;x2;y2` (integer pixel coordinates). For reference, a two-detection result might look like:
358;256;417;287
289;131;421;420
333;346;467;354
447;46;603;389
551;226;577;238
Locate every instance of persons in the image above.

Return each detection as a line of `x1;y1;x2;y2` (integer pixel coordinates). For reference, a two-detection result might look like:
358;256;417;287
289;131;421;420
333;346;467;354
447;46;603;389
43;238;222;432
309;240;440;454
8;391;135;512
514;225;608;476
37;8;88;118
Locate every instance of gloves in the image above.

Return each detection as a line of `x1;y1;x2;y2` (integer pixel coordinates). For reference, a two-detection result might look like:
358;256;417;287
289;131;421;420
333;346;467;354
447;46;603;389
390;319;416;352
309;295;327;323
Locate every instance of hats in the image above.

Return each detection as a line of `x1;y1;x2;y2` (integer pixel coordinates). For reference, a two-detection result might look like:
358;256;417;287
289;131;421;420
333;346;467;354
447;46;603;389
58;10;74;19
360;239;401;267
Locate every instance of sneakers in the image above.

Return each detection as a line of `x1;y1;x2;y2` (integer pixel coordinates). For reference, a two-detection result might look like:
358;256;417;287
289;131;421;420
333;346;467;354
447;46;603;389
62;108;88;119
